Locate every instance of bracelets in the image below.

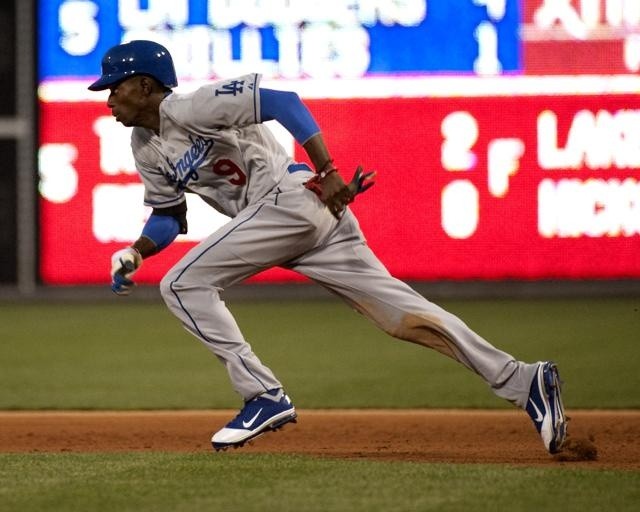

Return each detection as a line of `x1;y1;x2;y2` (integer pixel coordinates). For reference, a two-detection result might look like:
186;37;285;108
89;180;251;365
317;156;334;173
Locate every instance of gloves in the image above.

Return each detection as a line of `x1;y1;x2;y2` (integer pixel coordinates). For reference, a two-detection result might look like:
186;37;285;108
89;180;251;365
340;163;376;206
109;246;141;296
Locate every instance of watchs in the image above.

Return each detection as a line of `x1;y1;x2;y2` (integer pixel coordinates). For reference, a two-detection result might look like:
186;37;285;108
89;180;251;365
319;164;337;180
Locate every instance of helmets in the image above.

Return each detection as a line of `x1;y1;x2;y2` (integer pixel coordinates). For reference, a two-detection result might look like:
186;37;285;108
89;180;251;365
87;40;179;92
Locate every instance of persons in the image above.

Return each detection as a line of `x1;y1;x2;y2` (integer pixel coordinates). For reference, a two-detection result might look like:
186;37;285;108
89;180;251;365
87;40;568;455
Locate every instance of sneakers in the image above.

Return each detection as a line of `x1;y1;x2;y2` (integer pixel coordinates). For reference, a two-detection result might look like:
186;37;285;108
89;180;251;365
525;361;566;454
209;387;297;451
